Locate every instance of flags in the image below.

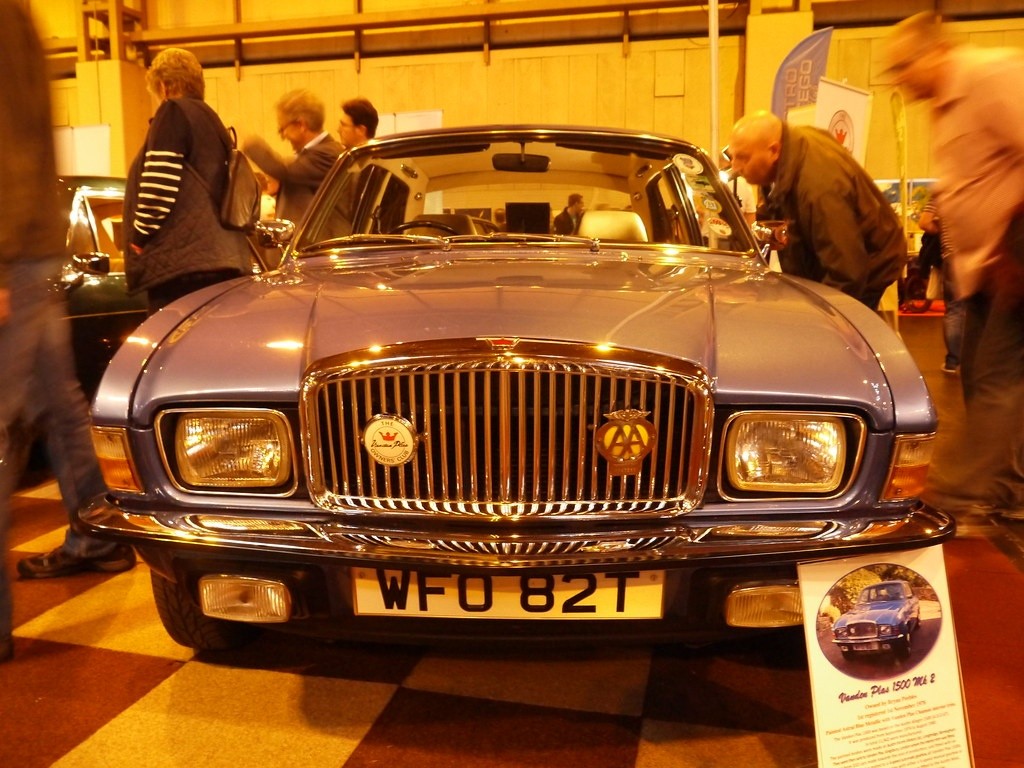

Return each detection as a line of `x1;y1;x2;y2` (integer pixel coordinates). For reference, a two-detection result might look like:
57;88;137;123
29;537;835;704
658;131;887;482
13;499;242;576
874;179;903;219
811;77;874;168
905;179;937;233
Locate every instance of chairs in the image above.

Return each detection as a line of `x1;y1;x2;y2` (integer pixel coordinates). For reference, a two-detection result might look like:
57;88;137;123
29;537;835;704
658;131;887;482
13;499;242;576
577;209;648;243
407;214;477;240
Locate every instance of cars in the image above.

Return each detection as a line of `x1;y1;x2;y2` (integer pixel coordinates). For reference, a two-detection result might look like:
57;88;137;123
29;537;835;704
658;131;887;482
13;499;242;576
0;172;266;490
80;119;949;666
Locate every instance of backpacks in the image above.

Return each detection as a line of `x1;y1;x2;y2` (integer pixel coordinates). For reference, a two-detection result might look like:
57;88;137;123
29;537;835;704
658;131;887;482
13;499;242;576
182;126;260;236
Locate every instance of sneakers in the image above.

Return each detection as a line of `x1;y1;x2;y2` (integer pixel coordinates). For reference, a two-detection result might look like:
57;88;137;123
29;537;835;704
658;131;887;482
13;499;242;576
18;526;135;580
940;352;960;373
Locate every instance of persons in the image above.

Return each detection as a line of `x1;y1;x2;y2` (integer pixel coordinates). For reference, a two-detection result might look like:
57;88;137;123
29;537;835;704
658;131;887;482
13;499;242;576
731;110;907;310
339;98;379;148
241;88;351;250
918;194;967;373
756;184;788;273
0;0;136;661
554;193;584;235
124;47;253;318
888;13;1024;522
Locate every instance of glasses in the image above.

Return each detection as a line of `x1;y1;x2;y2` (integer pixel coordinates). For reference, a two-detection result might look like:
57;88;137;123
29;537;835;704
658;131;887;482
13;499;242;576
885;47;936;76
278;119;297;134
